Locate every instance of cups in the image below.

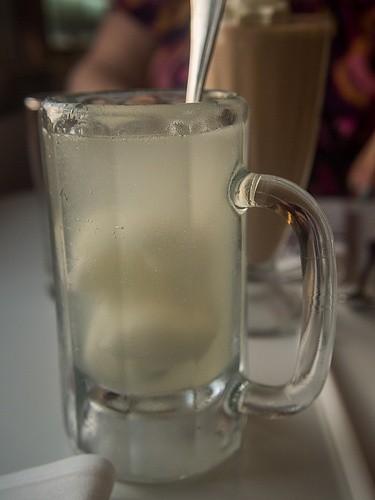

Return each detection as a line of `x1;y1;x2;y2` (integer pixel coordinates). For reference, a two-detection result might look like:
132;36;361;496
201;0;334;336
35;86;337;484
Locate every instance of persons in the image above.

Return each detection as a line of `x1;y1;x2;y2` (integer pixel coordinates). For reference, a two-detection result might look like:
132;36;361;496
63;0;375;196
